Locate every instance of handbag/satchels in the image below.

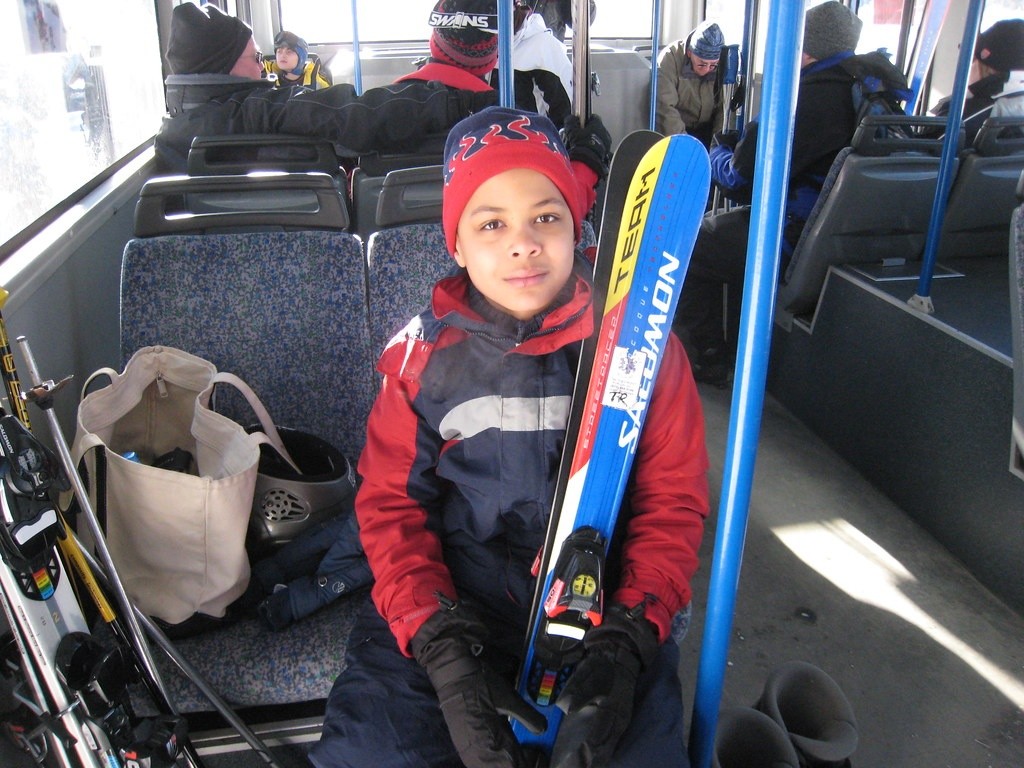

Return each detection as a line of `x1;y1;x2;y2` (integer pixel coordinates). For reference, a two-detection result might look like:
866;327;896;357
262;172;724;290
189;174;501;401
60;345;302;638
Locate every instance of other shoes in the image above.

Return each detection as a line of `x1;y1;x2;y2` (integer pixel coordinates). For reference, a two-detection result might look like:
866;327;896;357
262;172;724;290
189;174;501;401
677;336;732;390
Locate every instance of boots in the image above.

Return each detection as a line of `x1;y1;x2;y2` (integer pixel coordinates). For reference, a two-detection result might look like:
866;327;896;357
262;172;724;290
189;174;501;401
711;661;859;768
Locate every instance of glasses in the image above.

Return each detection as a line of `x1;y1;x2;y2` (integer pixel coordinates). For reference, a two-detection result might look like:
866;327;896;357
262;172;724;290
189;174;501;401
274;31;299;46
691;53;718;70
237;52;262;64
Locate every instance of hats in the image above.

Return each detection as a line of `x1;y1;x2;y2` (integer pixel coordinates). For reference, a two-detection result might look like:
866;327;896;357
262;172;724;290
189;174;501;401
443;106;581;258
274;36;308;74
803;1;862;60
689;20;725;60
428;0;499;77
974;19;1024;70
165;2;252;74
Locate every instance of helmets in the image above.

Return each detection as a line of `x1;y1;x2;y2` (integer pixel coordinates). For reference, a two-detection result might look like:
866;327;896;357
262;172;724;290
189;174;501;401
243;423;360;548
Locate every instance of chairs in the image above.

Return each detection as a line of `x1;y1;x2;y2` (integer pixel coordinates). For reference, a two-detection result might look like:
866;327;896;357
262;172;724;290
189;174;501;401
112;131;596;760
773;114;968;309
927;110;1024;258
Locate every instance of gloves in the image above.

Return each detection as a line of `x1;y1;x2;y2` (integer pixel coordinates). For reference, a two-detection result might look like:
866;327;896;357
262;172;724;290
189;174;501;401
802;69;915;192
409;590;548;768
548;598;658;768
564;114;612;188
710;128;739;150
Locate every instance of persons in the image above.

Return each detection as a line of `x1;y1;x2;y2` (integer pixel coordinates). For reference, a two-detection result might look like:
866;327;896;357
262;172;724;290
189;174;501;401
155;0;499;174
354;0;611;215
920;18;1024;142
687;0;915;381
315;107;709;768
654;22;737;134
263;32;332;92
485;0;573;132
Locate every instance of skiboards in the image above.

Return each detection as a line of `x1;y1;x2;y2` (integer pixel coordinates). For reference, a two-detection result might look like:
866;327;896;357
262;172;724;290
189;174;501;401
0;401;189;768
502;125;715;752
